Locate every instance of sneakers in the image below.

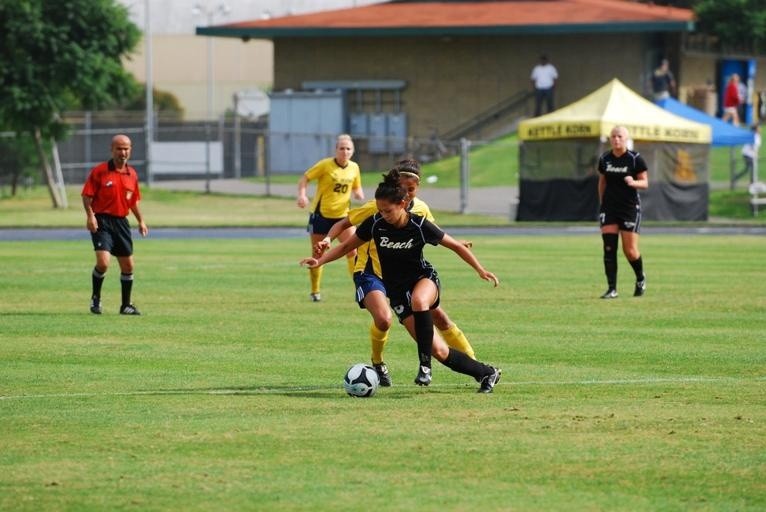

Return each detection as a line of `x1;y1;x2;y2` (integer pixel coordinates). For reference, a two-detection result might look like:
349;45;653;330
414;365;432;386
120;306;140;315
478;366;502;393
90;295;102;314
372;360;391;386
600;290;617;298
634;277;645;296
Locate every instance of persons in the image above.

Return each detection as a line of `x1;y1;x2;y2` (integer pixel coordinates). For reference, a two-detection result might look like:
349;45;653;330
298;166;503;394
730;125;762;185
80;133;149;316
312;157;478;388
595;123;650;300
297;131;367;303
650;57;678;102
720;72;742;126
528;52;560;118
737;81;748;123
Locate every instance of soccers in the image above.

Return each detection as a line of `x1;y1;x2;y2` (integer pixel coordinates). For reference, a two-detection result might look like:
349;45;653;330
344;363;379;398
